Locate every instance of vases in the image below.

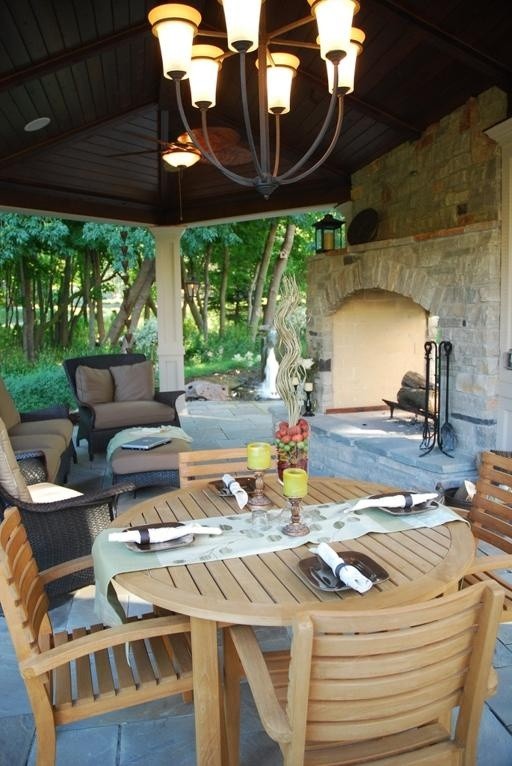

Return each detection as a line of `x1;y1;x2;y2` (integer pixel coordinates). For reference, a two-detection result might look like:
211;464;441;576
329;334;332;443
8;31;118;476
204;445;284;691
276;419;311;486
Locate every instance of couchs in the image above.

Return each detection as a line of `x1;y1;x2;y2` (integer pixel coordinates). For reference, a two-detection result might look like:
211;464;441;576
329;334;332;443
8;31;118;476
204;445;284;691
0;377;77;486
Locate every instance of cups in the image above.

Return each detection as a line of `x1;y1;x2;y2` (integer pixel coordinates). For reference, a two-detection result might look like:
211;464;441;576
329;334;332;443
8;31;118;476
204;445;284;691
251;510;269;539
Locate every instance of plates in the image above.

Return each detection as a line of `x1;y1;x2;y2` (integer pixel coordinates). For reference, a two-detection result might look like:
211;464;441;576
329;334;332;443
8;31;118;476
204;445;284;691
122;521;192;555
369;492;440;516
208;477;265;497
299;550;388;591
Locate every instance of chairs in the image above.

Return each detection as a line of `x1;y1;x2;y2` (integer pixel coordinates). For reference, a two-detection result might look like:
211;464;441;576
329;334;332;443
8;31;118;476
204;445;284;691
448;452;512;625
61;351;185;461
225;579;504;765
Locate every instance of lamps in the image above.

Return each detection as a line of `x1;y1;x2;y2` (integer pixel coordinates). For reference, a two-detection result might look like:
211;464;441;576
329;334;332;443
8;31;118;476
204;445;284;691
142;0;370;200
311;214;346;254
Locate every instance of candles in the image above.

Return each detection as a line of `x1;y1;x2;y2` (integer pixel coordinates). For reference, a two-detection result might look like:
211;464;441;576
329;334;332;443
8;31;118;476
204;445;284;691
247;440;272;470
283;465;309;498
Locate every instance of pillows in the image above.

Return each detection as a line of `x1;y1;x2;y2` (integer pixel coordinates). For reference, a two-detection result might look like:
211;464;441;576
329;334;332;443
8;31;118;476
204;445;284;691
74;360;157;405
